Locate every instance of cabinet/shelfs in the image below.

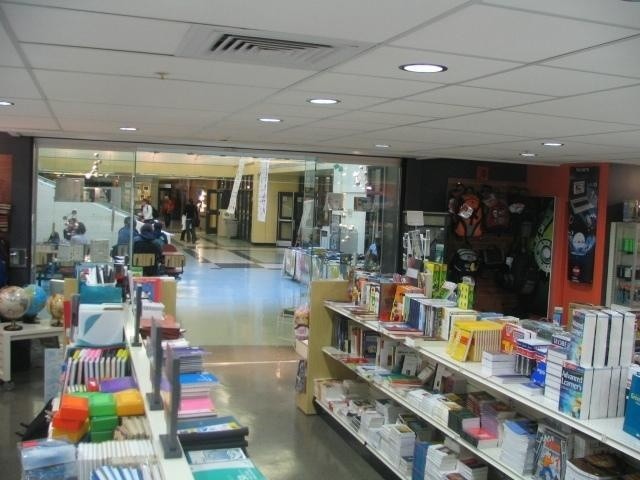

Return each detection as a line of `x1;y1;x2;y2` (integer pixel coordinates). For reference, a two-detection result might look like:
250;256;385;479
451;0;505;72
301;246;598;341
319;299;640;476
604;220;640;312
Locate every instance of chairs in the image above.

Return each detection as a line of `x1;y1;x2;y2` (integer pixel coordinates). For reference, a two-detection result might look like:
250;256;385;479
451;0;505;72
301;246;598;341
32;237;186;279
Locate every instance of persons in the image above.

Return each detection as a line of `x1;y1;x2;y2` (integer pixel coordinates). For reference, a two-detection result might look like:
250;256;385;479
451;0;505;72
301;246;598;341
136;199;154;224
133;222;162;276
49;218;90;245
153;221;168;245
117;217;139;254
452;240;483;280
160;195;175;231
178;206;197;241
183;198;200;243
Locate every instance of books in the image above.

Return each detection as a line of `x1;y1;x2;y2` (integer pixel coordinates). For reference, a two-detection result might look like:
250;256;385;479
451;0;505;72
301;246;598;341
293;258;640;480
15;264;268;479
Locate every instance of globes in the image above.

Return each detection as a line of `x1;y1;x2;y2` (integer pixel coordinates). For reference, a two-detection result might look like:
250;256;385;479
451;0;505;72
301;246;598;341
0;285;31;331
46;293;67;327
22;284;48;324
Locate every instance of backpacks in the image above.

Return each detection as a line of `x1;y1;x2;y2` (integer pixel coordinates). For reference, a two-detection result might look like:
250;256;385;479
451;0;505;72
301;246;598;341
448;182;486;242
445;241;480;288
477;185;535;234
475;244;510;291
496;243;541;298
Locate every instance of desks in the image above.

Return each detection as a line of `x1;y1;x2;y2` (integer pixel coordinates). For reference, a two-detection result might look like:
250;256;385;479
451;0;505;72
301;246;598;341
2;317;65;387
283;246;369;288
